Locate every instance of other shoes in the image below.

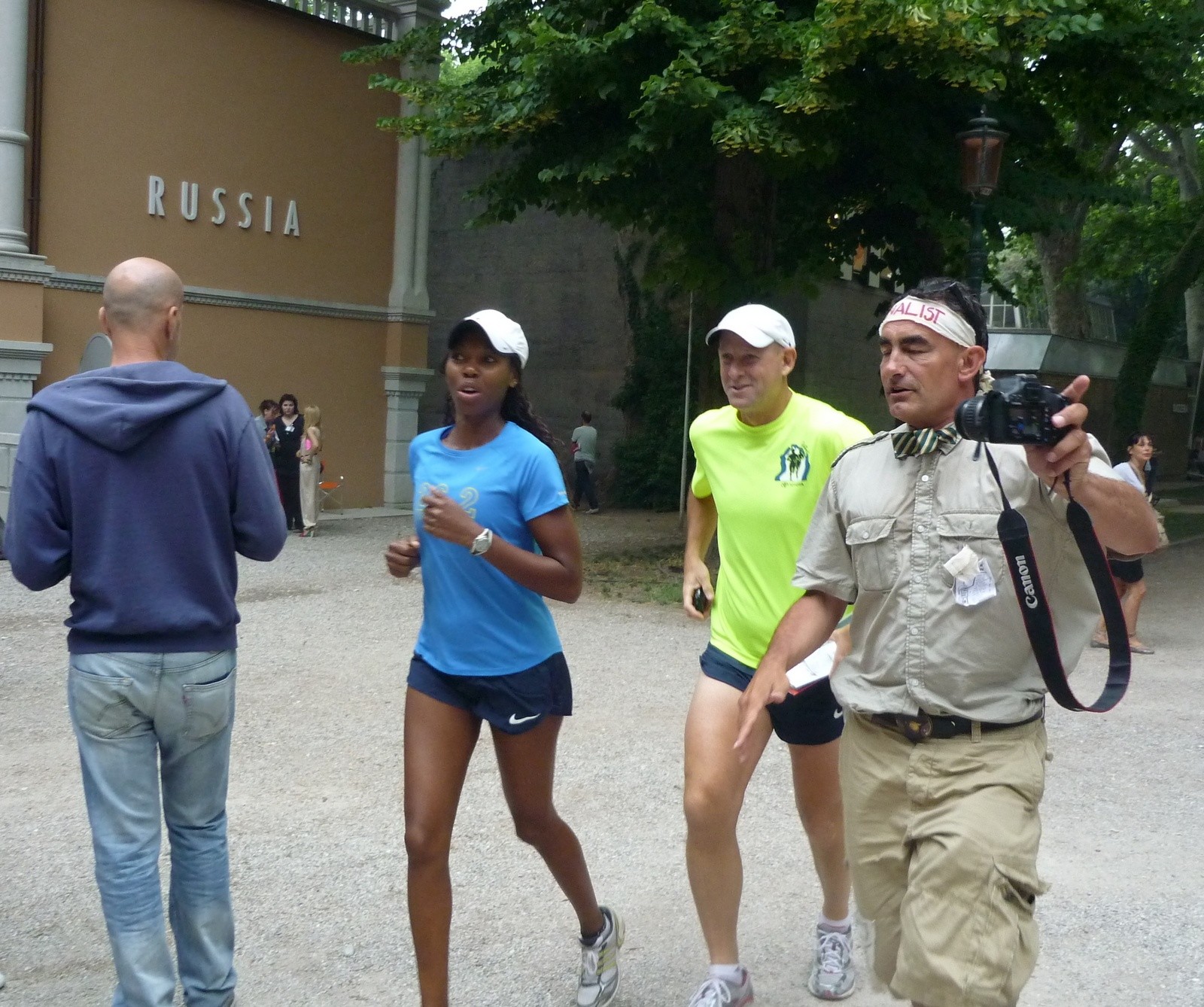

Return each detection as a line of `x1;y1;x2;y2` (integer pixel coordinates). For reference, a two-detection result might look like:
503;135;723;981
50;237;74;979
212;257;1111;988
585;509;599;514
1090;639;1109;648
568;503;577;510
1130;645;1154;654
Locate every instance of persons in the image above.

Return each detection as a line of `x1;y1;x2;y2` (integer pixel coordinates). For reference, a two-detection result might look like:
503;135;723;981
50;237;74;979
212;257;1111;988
571;410;600;514
1090;430;1169;654
9;254;287;1007
684;302;873;1007
253;393;304;531
296;404;322;537
729;277;1161;1007
383;310;626;1007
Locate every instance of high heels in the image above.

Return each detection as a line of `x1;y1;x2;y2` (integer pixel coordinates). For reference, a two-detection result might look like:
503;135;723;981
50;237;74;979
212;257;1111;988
300;525;315;538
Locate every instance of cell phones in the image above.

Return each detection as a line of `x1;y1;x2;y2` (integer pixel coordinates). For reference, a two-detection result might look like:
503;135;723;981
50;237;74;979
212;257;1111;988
693;589;707;613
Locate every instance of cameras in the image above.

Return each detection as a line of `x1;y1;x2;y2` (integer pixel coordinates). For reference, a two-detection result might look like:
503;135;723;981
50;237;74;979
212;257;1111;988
953;374;1073;444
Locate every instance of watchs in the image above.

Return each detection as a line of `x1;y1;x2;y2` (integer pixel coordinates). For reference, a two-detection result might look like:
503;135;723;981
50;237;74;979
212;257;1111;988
471;526;492;558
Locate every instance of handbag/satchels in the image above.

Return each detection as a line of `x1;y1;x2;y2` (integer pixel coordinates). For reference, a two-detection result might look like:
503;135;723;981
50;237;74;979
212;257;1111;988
1153;507;1170;548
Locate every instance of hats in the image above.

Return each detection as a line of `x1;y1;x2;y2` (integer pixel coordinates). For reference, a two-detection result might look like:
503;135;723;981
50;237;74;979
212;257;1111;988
705;304;796;352
448;308;530;370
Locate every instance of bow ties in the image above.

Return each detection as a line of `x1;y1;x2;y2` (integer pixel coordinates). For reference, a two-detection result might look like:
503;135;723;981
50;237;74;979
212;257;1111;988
890;427;956;458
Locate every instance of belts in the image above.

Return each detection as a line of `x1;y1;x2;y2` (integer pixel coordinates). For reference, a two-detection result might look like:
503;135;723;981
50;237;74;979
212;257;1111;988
858;706;1042;738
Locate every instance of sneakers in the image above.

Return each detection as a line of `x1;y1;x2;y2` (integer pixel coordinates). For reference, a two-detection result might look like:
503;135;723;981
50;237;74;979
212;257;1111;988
689;963;752;1007
577;907;625;1007
807;923;856;1000
